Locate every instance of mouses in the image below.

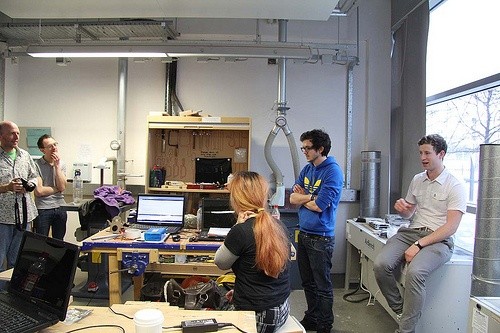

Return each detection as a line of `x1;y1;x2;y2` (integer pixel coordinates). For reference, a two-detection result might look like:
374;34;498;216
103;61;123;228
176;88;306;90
172;234;181;242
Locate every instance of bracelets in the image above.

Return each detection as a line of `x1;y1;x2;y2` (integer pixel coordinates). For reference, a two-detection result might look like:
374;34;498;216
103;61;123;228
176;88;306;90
309;194;315;201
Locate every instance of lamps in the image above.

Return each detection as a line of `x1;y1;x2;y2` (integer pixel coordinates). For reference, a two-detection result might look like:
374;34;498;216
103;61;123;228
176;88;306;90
26;44;312;59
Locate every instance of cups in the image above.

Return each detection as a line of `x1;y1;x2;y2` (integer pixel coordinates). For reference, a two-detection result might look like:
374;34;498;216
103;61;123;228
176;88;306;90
132;308;164;333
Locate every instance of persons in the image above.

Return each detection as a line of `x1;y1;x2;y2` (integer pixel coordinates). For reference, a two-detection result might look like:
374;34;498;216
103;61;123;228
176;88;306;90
374;134;470;332
214;171;293;333
0;120;67;272
289;130;344;333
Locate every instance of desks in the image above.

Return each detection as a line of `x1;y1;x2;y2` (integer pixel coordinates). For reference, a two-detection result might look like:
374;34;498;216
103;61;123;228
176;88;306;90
344;219;473;333
38;306;257;333
82;223;239;307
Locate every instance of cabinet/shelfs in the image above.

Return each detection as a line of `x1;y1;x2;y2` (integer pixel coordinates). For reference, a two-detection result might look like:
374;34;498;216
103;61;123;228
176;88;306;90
145;112;252;226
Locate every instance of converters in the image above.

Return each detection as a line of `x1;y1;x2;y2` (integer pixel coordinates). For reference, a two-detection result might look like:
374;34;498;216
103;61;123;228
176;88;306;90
181;319;218;333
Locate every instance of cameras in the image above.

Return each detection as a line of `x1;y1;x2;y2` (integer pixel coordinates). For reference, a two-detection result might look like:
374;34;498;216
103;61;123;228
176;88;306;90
17;178;36;193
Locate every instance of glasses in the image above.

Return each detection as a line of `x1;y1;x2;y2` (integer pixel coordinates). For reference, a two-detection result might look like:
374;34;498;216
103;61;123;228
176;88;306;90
40;141;58;150
300;146;314;152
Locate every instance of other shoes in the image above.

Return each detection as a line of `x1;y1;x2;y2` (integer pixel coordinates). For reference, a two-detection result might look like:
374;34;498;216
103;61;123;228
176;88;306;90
299;319;333;333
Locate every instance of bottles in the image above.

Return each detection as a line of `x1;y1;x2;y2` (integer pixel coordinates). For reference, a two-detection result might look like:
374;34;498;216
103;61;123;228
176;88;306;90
196;206;202;230
150;165;163;187
72;170;83;203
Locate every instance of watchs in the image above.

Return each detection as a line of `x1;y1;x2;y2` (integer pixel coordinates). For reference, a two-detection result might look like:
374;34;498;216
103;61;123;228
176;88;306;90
414;241;423;250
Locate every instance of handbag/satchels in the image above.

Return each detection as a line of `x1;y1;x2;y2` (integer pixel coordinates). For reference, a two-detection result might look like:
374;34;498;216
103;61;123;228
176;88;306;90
170;282;220;310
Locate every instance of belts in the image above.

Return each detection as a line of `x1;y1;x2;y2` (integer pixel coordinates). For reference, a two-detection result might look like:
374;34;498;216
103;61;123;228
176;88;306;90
415;227;434;233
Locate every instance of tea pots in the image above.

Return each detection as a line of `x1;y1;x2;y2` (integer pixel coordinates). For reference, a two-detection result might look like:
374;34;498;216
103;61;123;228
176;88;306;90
107;216;123;233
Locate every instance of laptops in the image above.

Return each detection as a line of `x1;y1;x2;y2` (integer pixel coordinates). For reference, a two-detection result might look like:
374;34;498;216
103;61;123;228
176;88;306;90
0;231;81;333
129;194;185;234
199;199;236;241
